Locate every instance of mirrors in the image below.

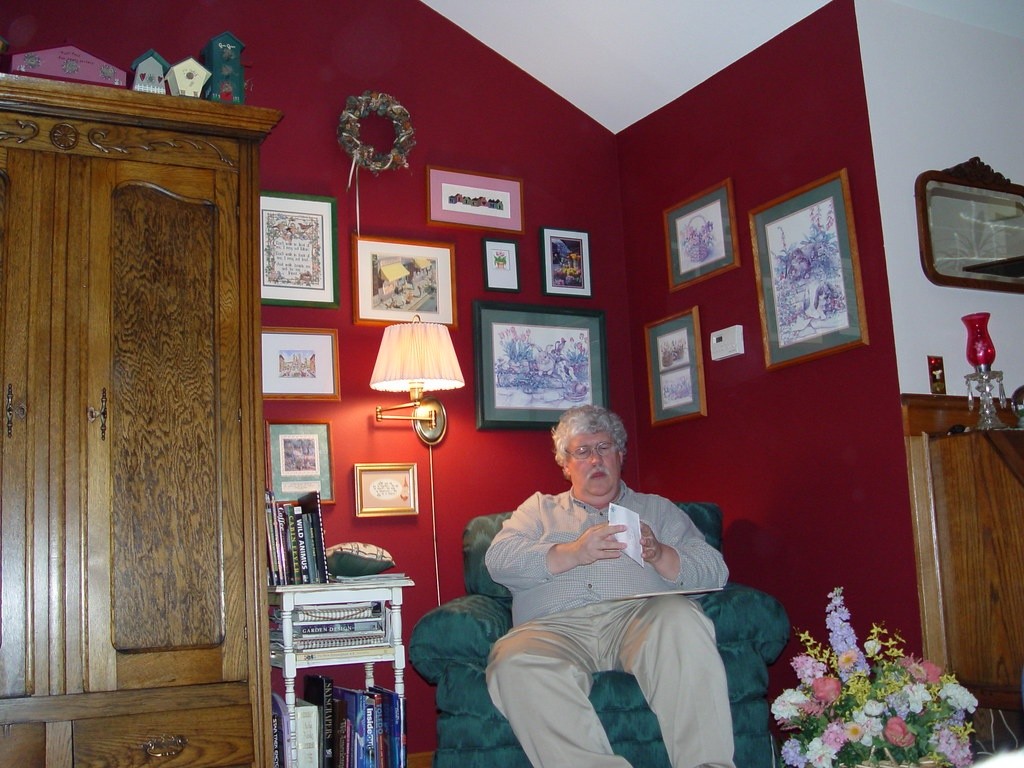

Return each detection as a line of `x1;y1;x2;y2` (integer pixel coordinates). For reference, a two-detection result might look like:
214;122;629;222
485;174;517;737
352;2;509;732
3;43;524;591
915;163;1024;294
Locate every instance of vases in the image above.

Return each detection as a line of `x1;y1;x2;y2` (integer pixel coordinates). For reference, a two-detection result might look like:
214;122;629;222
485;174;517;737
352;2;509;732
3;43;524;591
962;312;997;368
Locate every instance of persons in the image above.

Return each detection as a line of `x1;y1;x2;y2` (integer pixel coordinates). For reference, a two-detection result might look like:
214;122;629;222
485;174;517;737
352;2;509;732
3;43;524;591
484;403;735;768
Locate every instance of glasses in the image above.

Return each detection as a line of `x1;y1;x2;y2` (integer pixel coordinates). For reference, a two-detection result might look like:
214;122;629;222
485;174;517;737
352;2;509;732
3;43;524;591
564;442;619;459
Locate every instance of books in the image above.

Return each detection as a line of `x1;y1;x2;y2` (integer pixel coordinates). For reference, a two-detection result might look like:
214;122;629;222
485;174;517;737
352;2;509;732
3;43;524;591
264;490;330;587
271;674;408;768
268;603;396;662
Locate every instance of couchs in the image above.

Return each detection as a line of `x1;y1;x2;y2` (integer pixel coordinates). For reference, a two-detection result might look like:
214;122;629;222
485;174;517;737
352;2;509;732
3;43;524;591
408;500;791;768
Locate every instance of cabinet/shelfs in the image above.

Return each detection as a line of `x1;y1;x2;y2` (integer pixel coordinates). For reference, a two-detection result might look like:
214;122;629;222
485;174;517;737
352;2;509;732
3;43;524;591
1;69;286;768
266;575;414;768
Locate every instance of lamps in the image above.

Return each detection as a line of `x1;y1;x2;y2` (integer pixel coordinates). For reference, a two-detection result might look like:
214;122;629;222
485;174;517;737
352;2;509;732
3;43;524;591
369;315;464;446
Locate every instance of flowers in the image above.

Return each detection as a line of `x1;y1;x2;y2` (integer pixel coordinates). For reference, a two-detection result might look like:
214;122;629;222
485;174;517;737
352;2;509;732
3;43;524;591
772;587;978;768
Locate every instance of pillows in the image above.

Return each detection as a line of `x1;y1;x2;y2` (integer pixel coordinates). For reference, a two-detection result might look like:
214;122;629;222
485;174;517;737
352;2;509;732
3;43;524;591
325;542;395;576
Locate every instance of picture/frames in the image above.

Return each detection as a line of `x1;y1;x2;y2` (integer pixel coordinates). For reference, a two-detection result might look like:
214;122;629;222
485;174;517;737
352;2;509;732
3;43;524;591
643;304;707;426
351;233;457;329
481;236;520;292
259;189;340;308
661;177;741;293
261;326;341;402
426;164;526;237
472;298;610;430
541;225;593;299
746;166;870;370
264;418;336;506
354;462;418;518
